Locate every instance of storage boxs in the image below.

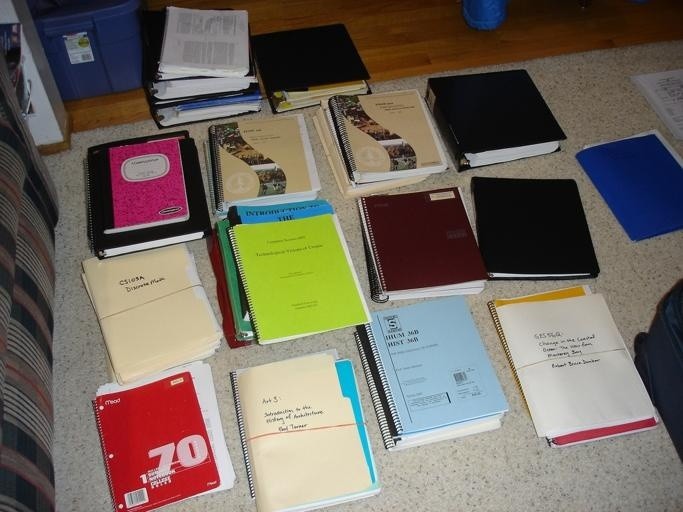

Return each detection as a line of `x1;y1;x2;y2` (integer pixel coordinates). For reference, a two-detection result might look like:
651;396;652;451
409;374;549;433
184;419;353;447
38;0;146;101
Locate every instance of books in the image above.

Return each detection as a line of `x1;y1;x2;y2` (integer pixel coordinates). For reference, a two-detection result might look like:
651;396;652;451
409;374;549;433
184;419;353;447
575;129;682;241
143;7;263;128
90;352;380;512
316;70;658;450
0;22;21;77
252;23;370;112
84;113;371;386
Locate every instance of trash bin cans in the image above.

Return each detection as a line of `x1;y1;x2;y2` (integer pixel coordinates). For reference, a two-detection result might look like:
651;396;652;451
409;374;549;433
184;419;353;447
25;0;143;103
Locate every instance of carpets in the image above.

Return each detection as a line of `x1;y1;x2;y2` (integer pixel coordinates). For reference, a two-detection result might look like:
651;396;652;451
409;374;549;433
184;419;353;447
39;43;683;512
